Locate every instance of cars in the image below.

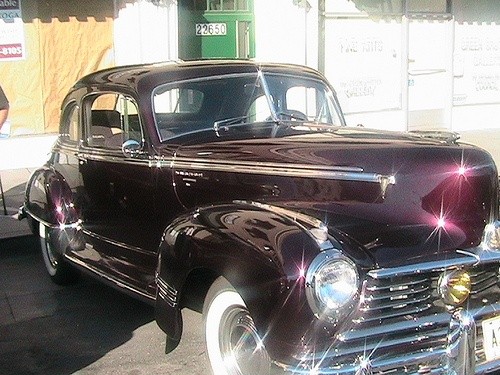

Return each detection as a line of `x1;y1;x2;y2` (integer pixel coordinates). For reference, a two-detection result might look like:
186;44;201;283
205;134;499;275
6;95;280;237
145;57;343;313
10;57;500;375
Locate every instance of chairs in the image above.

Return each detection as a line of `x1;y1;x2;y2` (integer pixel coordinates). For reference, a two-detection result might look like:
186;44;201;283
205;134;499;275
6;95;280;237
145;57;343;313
265;110;308;122
91;126;112;146
101;131;141;147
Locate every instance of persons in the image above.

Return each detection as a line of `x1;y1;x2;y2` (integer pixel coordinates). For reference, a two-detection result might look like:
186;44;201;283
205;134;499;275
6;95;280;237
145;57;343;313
0;85;9;130
